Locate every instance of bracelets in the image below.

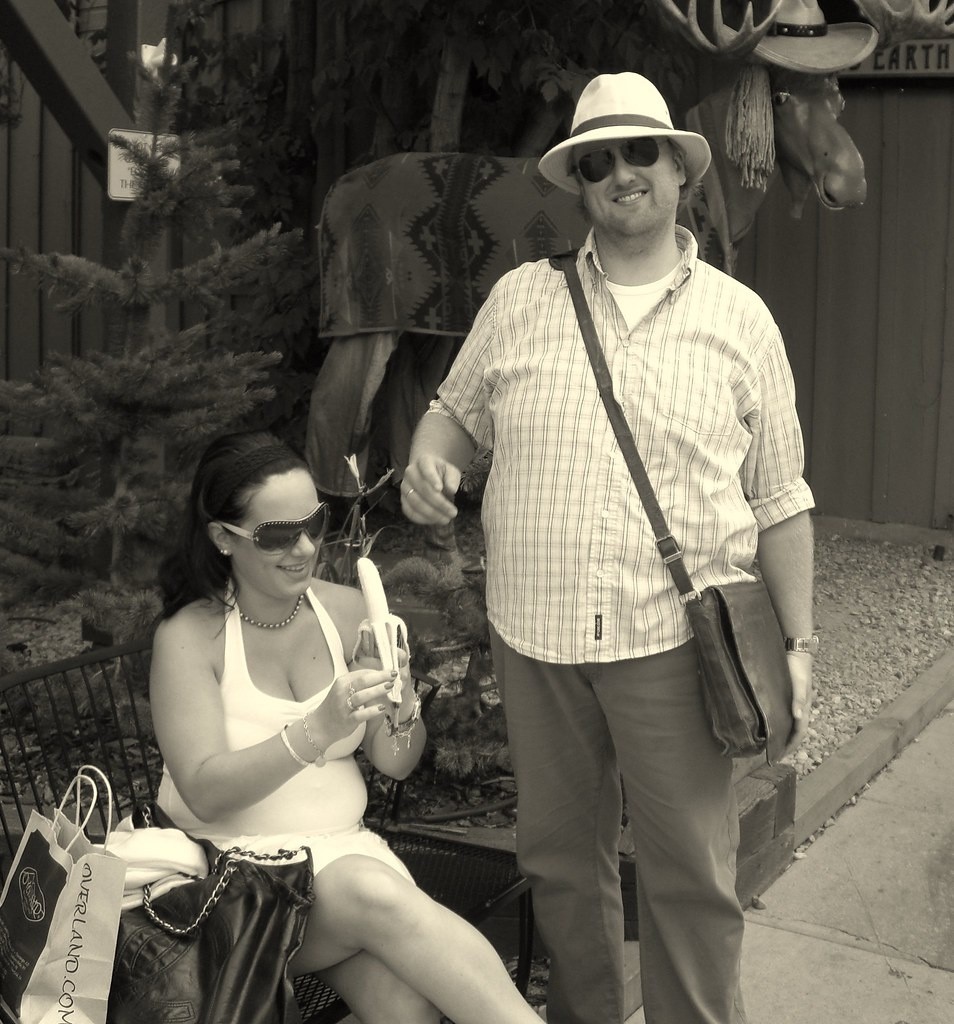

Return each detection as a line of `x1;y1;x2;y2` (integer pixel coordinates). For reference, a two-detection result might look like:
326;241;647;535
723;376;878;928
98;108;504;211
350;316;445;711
383;687;422;755
280;712;328;769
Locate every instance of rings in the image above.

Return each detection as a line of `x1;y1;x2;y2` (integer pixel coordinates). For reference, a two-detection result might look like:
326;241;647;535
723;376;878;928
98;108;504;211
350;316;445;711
405;488;415;497
346;682;356;711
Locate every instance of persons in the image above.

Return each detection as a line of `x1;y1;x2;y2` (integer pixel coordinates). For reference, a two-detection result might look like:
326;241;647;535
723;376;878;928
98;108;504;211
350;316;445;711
151;429;551;1024
400;71;815;1024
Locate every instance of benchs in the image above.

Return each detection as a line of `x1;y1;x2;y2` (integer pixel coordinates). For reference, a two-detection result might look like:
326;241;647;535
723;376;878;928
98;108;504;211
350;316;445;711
0;628;537;1024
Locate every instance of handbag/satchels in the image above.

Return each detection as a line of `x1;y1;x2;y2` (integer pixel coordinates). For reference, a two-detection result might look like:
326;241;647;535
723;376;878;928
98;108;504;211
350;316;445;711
0;765;128;1024
107;802;314;1024
685;579;795;766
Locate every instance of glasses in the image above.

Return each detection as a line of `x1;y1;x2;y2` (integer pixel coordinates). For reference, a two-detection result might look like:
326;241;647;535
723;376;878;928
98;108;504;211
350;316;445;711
576;137;669;183
215;501;330;555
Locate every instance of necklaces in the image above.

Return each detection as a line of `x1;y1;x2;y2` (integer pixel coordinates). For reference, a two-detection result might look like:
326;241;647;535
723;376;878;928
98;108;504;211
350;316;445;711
240;594;304;629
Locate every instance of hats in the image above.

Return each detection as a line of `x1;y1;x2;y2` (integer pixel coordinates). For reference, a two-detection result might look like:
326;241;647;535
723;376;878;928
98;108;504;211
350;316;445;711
537;71;712;197
722;0;880;74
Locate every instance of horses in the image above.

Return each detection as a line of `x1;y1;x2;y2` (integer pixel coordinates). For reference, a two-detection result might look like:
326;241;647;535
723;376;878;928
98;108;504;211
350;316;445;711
301;50;867;590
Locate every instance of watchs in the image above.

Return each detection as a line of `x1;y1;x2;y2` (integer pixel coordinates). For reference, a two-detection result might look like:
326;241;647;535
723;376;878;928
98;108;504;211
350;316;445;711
784;635;818;658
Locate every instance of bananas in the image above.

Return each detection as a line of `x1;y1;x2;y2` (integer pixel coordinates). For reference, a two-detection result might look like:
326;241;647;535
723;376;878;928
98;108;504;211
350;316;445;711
356;556;411;700
